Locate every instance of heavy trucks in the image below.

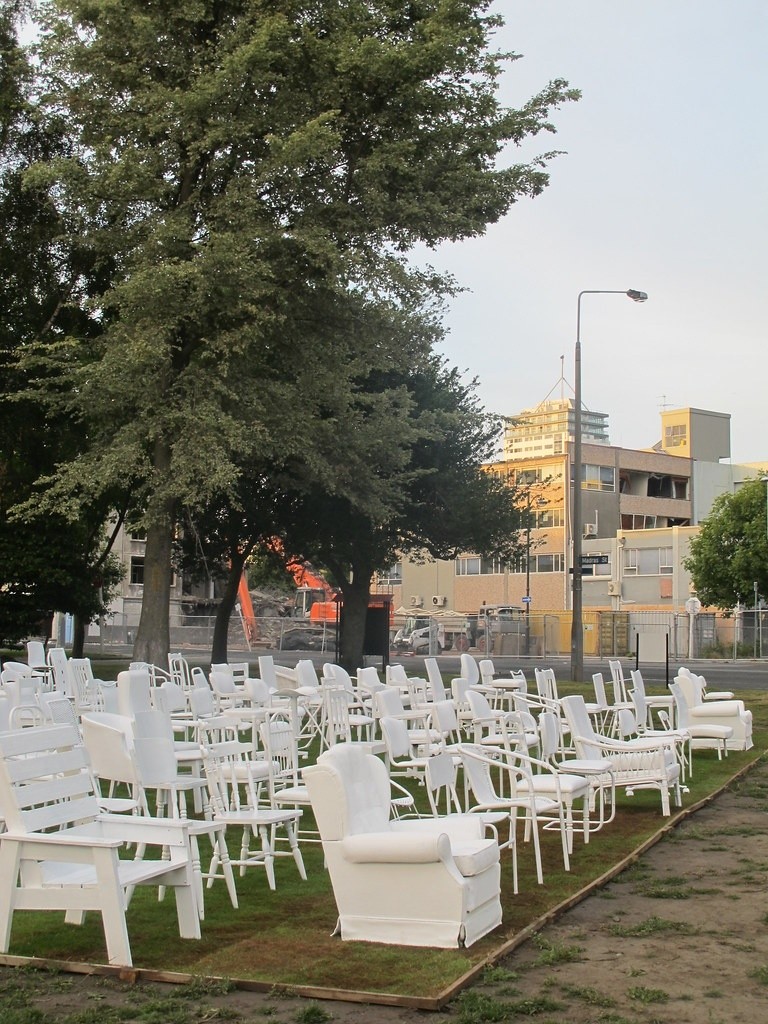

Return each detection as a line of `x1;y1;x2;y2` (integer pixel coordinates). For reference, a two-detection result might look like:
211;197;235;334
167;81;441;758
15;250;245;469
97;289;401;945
390;603;526;653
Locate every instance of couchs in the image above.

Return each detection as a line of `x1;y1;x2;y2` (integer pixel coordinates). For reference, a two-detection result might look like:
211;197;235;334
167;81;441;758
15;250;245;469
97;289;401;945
676;667;754;751
301;746;504;949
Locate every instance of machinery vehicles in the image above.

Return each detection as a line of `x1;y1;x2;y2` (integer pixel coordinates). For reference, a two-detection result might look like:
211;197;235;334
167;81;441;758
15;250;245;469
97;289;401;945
225;534;395;653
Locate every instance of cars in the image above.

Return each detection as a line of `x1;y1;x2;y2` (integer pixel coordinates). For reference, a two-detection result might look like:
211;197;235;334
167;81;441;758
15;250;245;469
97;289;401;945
275;627;339;652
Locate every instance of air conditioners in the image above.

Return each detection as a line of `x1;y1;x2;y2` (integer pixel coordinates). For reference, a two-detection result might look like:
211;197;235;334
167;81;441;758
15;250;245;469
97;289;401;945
585;524;597;535
608;581;621;596
411;596;421;606
432;596;444;606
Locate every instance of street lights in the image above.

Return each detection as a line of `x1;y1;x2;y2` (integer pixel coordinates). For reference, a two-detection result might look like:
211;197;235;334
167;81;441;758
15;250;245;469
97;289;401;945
571;287;649;683
524;491;547;654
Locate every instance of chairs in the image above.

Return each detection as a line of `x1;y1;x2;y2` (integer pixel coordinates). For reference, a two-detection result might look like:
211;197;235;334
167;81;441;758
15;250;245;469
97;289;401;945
0;640;736;971
